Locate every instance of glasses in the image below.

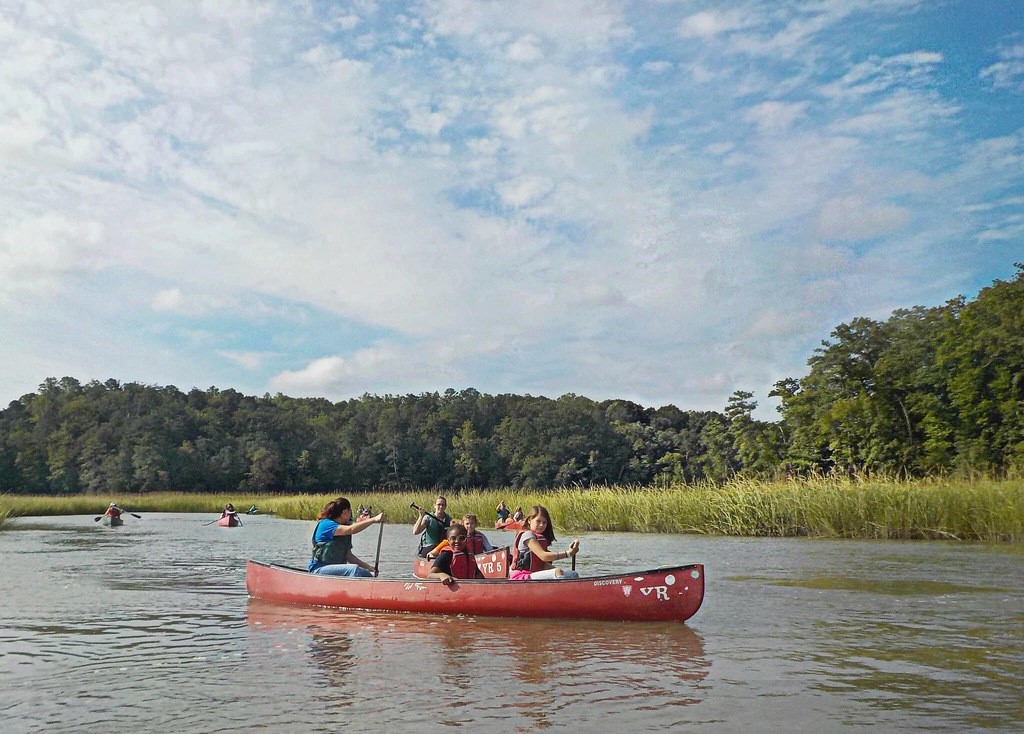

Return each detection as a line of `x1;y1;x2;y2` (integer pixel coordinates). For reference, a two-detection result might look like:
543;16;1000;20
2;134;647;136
450;535;468;542
436;504;445;506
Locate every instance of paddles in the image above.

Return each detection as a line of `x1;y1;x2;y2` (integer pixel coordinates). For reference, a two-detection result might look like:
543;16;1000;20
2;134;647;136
123;510;142;519
202;517;223;526
94;515;106;522
409;501;449;527
235;514;243;526
569;541;576;571
373;514;387;576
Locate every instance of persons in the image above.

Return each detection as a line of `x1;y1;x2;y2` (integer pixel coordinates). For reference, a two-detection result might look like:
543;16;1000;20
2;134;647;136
412;497;581;586
222;503;236;518
357;504;372;516
105;501;120;519
249;504;257;511
308;497;387;578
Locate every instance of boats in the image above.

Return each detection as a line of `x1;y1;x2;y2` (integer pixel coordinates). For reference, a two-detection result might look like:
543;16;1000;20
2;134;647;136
412;546;510;580
217;514;239;527
356;514;370;522
245;559;706;623
102;515;123;527
